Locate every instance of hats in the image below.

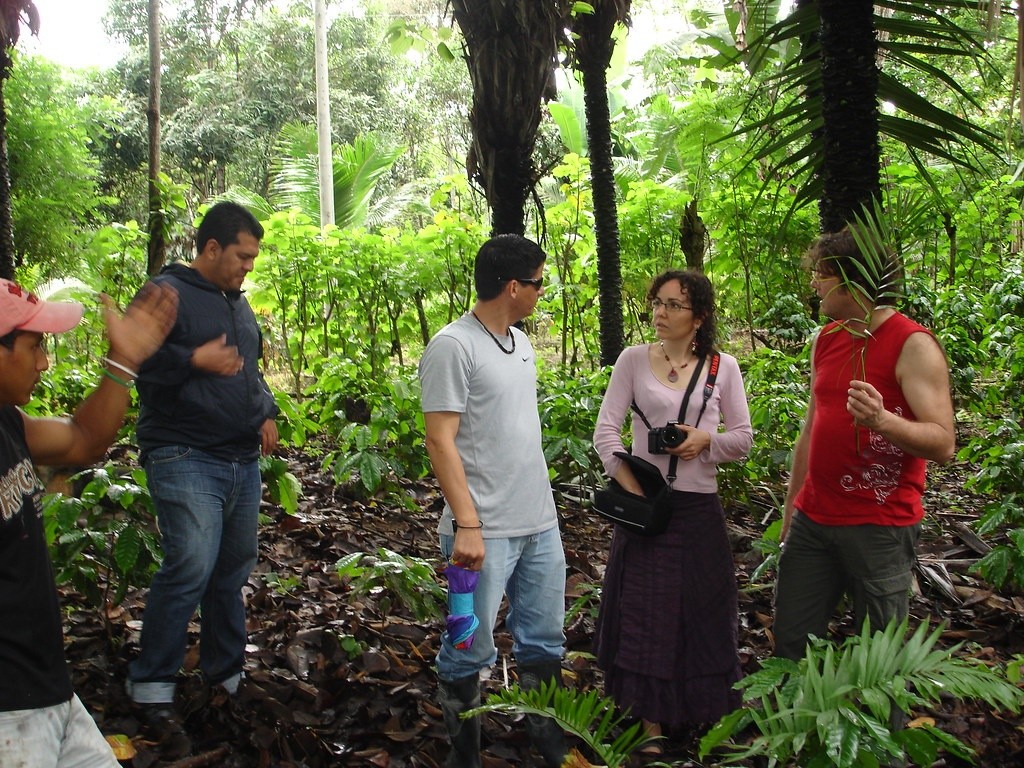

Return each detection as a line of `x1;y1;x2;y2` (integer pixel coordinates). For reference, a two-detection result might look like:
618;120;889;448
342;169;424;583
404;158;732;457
1;276;83;339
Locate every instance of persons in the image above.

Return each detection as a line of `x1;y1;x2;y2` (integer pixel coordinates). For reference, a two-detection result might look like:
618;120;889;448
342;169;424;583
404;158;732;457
418;234;573;768
593;270;754;756
0;279;179;768
125;202;280;758
771;231;955;689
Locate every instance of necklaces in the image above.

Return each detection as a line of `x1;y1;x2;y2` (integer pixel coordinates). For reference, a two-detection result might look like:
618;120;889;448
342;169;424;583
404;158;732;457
660;343;696;383
470;309;515;354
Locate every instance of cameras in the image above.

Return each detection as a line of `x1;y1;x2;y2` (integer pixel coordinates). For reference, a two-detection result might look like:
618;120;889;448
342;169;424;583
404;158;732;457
648;421;688;455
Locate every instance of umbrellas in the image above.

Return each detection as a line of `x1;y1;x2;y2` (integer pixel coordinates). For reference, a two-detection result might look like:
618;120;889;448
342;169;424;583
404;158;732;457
444;519;480;651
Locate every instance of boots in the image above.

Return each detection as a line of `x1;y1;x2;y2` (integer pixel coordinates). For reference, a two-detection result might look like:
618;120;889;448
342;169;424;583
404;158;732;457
517;658;570;768
435;672;481;768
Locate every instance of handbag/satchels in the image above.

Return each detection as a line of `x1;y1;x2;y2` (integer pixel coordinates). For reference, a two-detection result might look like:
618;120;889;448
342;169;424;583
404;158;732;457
592;450;673;534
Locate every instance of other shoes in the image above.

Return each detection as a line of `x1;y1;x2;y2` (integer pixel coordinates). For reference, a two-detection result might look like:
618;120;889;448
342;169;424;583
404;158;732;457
637;735;665;757
144;703;189;758
211;678;267;700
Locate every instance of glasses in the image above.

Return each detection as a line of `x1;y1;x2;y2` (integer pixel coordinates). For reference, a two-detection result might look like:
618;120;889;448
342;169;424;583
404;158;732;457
650;297;696;313
810;268;840;283
497;275;543;290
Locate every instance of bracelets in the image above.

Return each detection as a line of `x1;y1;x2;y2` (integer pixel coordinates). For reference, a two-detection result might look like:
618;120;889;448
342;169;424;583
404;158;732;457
457;520;483;529
104;370;134;388
104;359;138;378
